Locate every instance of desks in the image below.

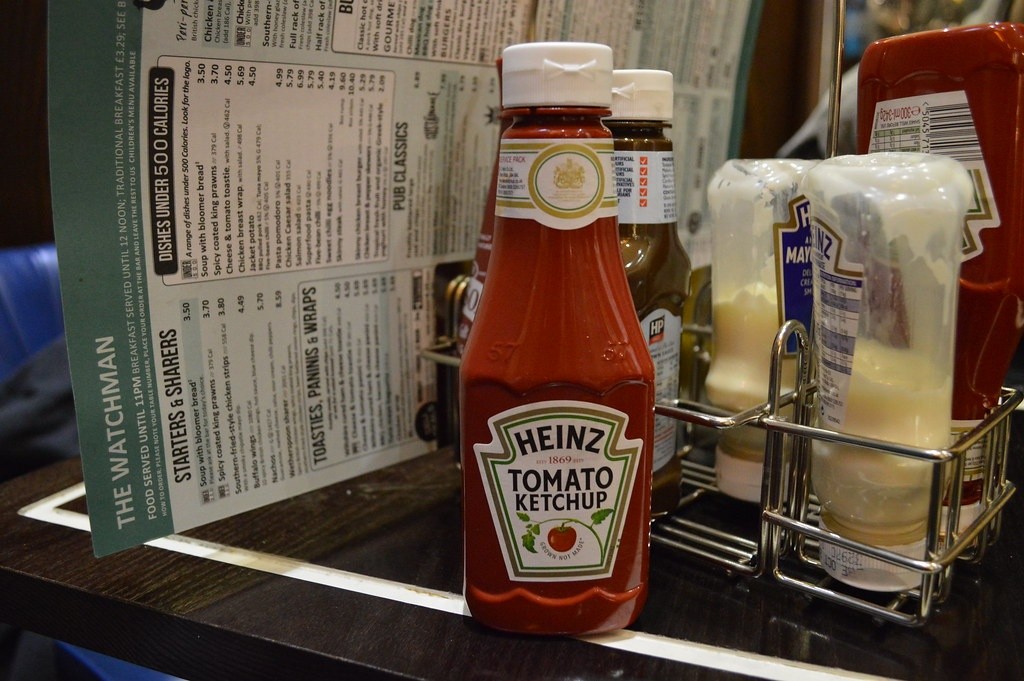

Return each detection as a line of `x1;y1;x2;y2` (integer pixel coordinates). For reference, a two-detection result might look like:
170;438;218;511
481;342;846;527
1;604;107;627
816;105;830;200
0;409;1024;681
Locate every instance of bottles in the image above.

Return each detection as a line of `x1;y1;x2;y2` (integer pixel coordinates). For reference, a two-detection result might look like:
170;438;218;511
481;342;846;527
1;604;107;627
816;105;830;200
806;152;965;592
855;20;1024;539
458;40;653;635
704;158;820;505
458;59;506;357
604;69;692;512
